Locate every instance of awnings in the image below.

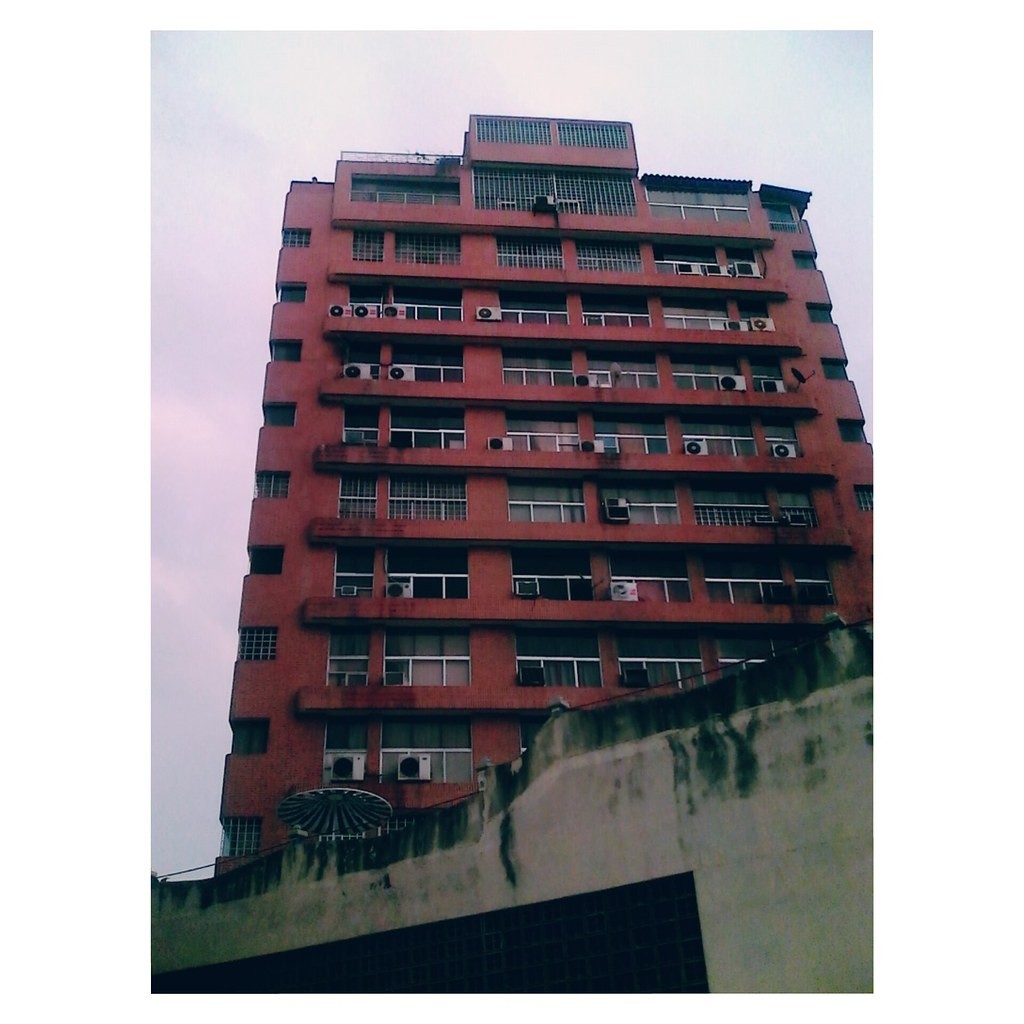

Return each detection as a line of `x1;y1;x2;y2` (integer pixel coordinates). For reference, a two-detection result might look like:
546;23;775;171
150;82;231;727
640;172;753;190
759;181;813;220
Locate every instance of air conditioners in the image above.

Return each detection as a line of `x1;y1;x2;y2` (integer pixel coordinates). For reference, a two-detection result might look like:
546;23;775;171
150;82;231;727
750;316;776;332
772;444;797;458
717;374;747;391
385;582;412;599
515;580;540;596
328;304;352;317
397;753;431;780
382;304;406;319
609;582;639;602
475;306;502;321
704;264;727;276
724;321;749;332
684;441;708;456
578;440;604;453
733;261;761;279
330;754;366;781
387;366;415;382
677;264;702;276
343;363;371;380
573;374;598;388
486;437;513;451
760;379;785;393
534;195;553;205
353;304;377;318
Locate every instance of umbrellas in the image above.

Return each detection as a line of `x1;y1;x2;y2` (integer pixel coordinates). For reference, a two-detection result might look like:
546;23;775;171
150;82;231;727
274;787;393;845
